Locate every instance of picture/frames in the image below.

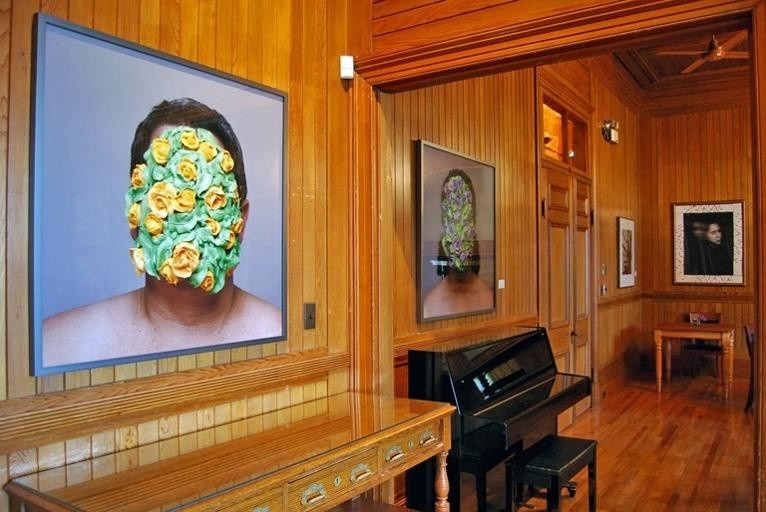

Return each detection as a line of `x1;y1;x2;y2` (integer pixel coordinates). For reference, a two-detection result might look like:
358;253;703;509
671;199;746;287
416;138;499;324
29;11;289;378
616;216;636;289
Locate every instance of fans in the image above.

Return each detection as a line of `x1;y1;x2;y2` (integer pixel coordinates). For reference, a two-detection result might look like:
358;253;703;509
656;29;749;73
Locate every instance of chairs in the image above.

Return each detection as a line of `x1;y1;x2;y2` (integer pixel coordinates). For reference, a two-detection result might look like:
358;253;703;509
744;322;754;413
682;312;723;378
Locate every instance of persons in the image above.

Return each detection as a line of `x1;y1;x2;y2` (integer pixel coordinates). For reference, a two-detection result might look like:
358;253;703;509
685;220;734;277
41;95;283;369
423;168;496;322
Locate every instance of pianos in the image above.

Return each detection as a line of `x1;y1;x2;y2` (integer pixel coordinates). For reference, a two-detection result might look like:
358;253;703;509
405;326;592;511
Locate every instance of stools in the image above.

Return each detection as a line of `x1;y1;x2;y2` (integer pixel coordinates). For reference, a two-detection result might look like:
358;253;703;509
503;433;598;512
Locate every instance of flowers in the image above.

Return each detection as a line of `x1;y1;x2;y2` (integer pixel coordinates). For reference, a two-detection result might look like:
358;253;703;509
123;126;242;296
440;176;476;272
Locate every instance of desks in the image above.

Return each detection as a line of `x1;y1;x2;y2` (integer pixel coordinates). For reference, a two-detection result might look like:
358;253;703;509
4;390;458;512
654;321;736;403
